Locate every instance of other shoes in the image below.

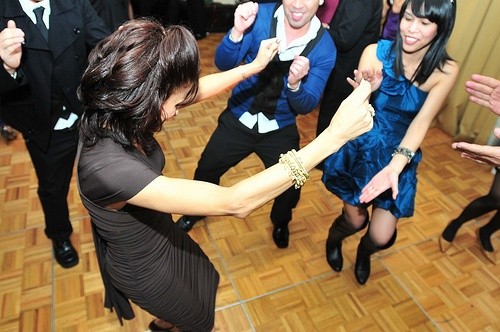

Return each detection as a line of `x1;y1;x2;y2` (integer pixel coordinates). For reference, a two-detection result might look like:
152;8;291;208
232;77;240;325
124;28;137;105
148;319;175;332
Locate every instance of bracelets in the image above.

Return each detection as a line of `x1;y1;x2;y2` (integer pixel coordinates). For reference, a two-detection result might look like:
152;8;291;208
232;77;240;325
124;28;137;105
292;149;309;179
279;152;299;189
288;151;307;183
390;146;415;163
285;151;303;186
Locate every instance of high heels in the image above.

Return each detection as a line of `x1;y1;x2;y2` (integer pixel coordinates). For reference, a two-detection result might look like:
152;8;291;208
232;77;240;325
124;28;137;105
476;227;497;264
440;220;456;253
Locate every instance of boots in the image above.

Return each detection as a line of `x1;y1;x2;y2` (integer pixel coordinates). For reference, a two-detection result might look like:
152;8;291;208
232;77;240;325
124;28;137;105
355;220;397;284
326;207;369;272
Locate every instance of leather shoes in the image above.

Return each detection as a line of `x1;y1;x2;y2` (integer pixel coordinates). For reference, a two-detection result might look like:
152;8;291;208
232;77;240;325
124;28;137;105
52;239;77;267
273;224;289;248
176;214;205;232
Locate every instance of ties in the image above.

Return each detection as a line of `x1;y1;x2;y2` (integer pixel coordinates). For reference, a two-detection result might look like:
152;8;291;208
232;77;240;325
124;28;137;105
34;6;71;119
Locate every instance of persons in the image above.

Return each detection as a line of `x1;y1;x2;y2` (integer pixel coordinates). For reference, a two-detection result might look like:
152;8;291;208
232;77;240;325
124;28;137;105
322;0;458;284
438;139;500;266
0;0;500;332
451;73;500;169
315;0;382;171
0;0;113;268
177;0;336;251
75;15;374;332
